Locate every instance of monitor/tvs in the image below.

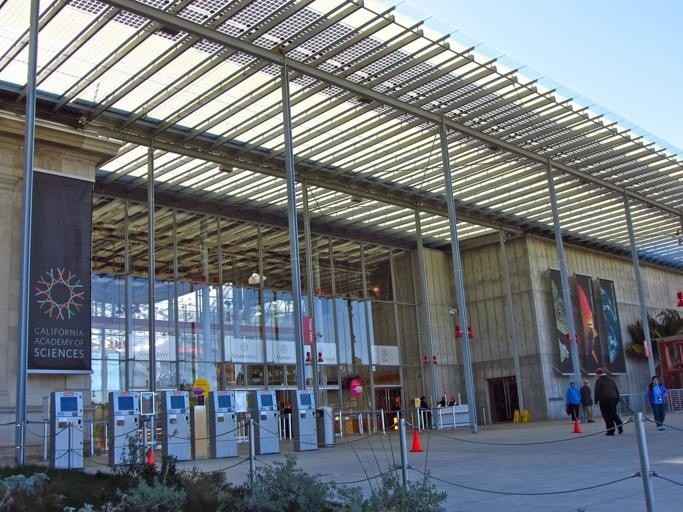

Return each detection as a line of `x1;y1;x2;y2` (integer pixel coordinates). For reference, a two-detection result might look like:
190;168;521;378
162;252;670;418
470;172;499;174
208;391;235;412
50;392;83;417
161;391;189;411
290;390;316;409
108;392;139;414
251;390;277;411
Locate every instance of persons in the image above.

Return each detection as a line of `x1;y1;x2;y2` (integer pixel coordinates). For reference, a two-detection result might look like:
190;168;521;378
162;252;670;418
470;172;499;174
283;403;292;438
447;396;458;406
580;380;595;423
420;396;430;428
566;382;582;423
594;368;623;436
437;396;445;407
646;376;668;431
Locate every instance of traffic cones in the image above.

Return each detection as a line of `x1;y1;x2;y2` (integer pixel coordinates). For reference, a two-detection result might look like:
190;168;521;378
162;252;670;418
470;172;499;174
147;446;156;463
410;432;424;453
573;417;583;433
